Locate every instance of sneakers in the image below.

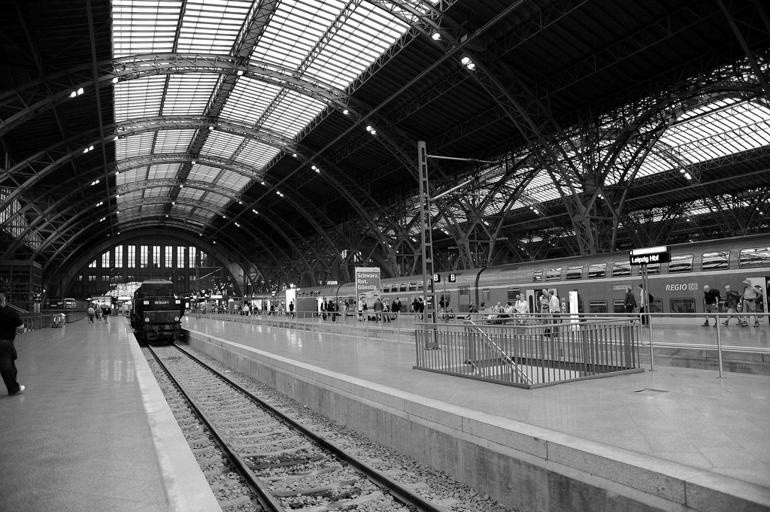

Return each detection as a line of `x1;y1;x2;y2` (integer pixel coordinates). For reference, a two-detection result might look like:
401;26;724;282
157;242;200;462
702;320;759;327
18;386;25;391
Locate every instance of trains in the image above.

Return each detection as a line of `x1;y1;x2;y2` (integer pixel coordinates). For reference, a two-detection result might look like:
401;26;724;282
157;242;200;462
266;225;770;317
129;276;187;347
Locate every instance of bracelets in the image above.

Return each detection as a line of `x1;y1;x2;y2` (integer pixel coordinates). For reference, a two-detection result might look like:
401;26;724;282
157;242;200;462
716;302;719;304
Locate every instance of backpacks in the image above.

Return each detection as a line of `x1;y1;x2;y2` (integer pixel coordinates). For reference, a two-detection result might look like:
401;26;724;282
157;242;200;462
710;289;721;303
732;291;740;303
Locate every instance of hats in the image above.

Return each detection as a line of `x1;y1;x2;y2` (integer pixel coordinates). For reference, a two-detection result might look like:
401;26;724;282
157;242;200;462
742;279;751;285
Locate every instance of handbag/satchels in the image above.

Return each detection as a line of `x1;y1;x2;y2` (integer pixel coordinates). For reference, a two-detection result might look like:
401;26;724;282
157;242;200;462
648;293;653;303
544;329;550;337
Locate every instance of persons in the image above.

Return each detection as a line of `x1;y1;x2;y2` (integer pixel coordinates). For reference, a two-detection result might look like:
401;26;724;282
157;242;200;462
758;287;764;312
637;283;651;326
701;284;720;327
741;279;763;328
623;288;637;323
538;289;551;336
572;288;587;322
238;293;530;326
0;292;28;396
721;285;744;325
547;289;561;338
86;302;111;326
